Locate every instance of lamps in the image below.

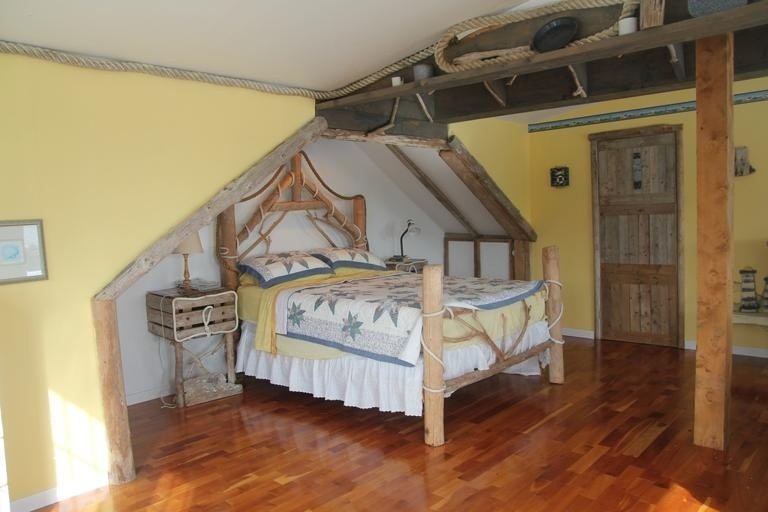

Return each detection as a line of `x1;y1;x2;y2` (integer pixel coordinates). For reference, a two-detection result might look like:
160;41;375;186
389;219;422;262
171;231;204;284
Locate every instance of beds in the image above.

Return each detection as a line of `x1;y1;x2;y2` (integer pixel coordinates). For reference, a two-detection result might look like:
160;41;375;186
216;150;566;447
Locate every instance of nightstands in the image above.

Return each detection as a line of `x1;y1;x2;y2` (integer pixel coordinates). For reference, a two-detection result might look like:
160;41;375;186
146;282;243;408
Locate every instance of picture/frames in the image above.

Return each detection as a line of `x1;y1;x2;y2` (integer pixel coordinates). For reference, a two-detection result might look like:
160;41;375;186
0;219;48;285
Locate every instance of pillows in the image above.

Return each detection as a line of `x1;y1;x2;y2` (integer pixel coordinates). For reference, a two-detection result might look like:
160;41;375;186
237;250;336;289
307;247;389;276
